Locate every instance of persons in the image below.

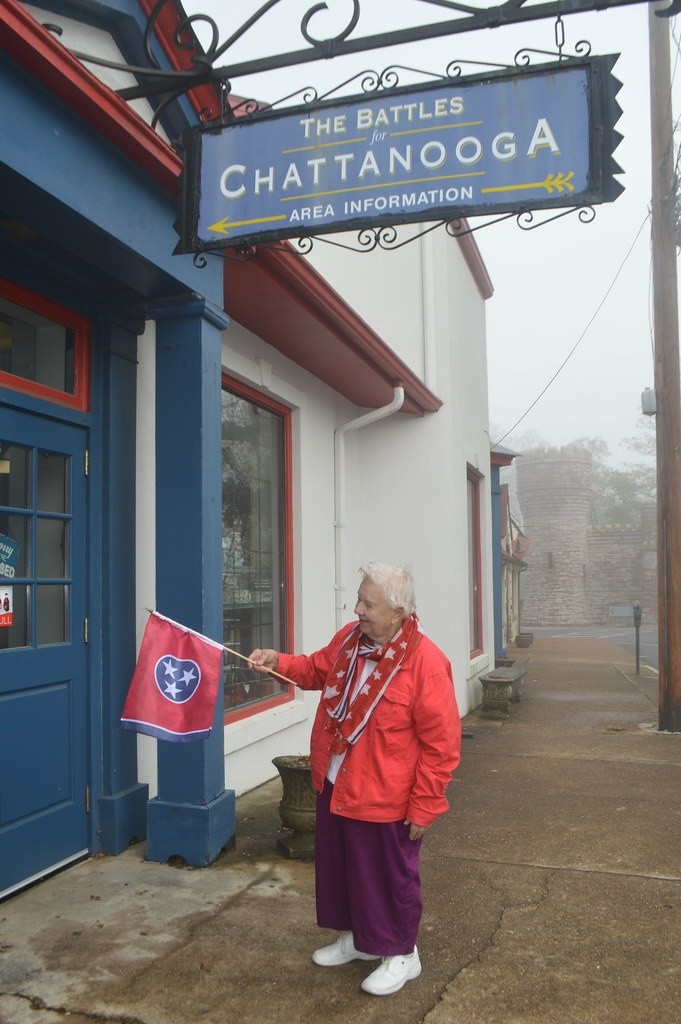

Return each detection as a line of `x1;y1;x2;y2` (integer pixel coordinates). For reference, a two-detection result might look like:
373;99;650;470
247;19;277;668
248;561;461;995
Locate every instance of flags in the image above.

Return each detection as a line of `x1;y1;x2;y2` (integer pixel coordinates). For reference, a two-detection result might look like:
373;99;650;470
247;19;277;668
120;615;222;744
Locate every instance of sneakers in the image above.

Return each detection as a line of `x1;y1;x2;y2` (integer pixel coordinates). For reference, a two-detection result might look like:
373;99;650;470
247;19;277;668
361;946;423;995
313;932;382;967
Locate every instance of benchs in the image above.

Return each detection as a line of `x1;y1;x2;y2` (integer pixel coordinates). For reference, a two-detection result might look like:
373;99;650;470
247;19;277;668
478;668;526;717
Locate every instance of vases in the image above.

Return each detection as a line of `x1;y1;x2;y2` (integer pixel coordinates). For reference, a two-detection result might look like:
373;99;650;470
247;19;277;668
272;756;315;857
515;632;533;647
